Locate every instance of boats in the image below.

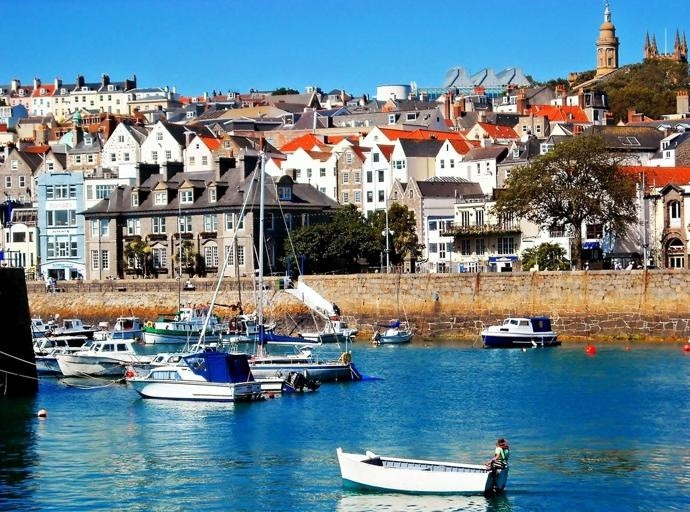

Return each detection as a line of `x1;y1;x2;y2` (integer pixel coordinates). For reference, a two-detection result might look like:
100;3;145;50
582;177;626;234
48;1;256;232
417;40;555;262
335;446;509;497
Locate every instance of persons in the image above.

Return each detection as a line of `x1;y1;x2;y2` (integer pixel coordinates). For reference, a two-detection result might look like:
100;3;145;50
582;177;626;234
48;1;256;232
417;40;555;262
486;439;508;470
